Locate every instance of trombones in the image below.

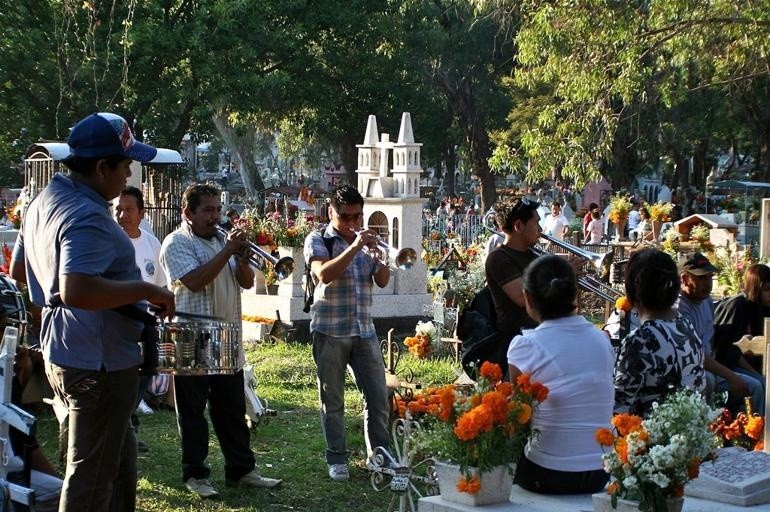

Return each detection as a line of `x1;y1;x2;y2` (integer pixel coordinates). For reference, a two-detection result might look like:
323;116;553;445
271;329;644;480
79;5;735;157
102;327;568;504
482;211;623;304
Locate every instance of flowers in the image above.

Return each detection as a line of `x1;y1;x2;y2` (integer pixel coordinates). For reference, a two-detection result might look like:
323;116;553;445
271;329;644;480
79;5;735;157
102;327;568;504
0;199;28;295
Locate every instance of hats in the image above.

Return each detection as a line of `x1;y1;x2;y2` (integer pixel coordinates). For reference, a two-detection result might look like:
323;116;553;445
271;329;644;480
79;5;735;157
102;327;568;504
68;107;158;165
680;250;723;279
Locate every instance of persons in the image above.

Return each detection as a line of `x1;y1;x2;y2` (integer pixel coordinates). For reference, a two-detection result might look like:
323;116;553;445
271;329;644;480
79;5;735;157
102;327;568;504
505;253;615;496
226;210;239;230
9;111;176;512
602;248;770;419
157;182;282;499
110;186;168;453
485;201;589;347
0;305;56;477
435;201;478;242
534;182;673;244
303;184;395;482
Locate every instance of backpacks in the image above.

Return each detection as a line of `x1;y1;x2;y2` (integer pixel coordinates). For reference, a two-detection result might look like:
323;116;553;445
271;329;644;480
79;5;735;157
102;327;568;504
455;248;526;383
302;226;335;313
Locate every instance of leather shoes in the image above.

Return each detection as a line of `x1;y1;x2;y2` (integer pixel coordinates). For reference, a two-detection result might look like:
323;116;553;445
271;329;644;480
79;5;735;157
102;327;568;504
185;477;221;498
224;468;283;489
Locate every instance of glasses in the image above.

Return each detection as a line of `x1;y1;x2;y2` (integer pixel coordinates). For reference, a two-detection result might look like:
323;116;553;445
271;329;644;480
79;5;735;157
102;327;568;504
337;210;363;222
509;195;531;231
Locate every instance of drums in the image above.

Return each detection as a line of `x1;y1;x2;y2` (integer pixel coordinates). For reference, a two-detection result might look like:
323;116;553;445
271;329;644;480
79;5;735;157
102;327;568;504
1;272;28;364
148;320;239;376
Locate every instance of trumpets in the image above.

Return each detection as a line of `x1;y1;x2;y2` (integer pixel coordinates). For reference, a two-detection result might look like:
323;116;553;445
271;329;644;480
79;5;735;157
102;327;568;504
348;227;417;271
213;223;295;281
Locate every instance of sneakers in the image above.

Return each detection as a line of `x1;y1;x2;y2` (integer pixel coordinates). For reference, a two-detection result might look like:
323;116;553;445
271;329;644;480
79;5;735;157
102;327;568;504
134;397;154;416
326;460;351;482
